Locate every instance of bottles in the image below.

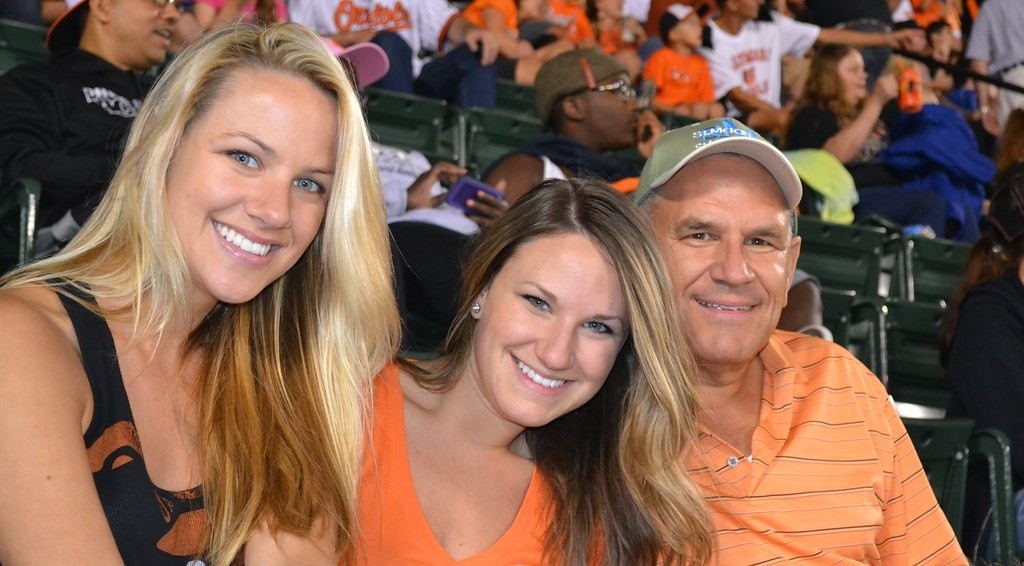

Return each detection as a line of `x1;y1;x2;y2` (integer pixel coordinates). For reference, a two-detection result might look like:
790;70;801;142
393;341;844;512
898;66;923;114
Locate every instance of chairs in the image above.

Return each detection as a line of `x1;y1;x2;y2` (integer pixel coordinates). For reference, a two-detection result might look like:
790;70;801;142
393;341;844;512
791;209;1024;566
358;72;543;187
0;179;41;275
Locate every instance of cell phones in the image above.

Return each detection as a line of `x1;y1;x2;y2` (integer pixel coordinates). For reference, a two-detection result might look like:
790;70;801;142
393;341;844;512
444;176;505;216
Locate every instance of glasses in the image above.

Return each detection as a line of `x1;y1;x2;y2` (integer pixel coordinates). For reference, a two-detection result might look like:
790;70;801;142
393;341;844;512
152;0;182;11
572;81;635;104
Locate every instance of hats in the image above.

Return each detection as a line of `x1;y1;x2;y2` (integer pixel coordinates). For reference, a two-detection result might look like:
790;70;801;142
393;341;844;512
44;0;91;57
658;0;712;43
535;48;628;122
633;118;803;239
316;34;389;89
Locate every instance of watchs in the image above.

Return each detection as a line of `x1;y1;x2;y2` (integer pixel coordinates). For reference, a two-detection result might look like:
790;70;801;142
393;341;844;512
980;105;989;113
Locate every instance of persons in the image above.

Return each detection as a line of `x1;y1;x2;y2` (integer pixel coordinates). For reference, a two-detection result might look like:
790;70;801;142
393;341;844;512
334;176;719;566
626;115;969;566
964;0;1023;139
0;0;183;262
0;25;366;566
36;1;1023;360
946;107;1024;566
